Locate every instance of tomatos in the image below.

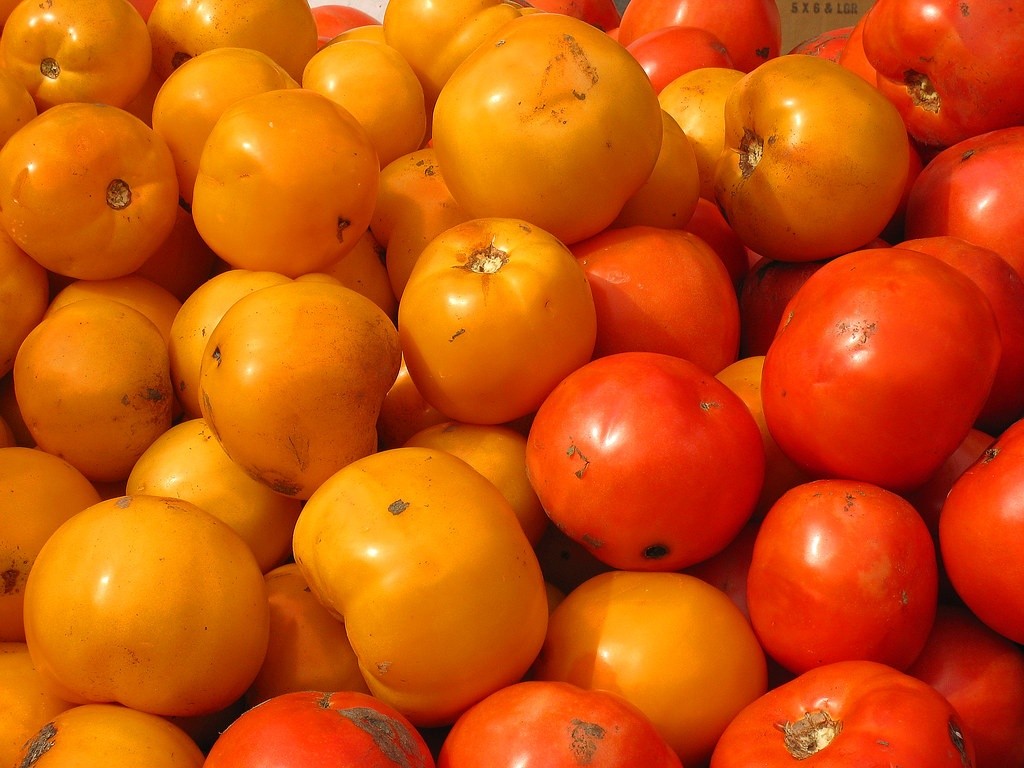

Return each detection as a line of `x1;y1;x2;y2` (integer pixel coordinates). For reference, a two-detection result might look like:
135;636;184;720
0;0;1024;768
